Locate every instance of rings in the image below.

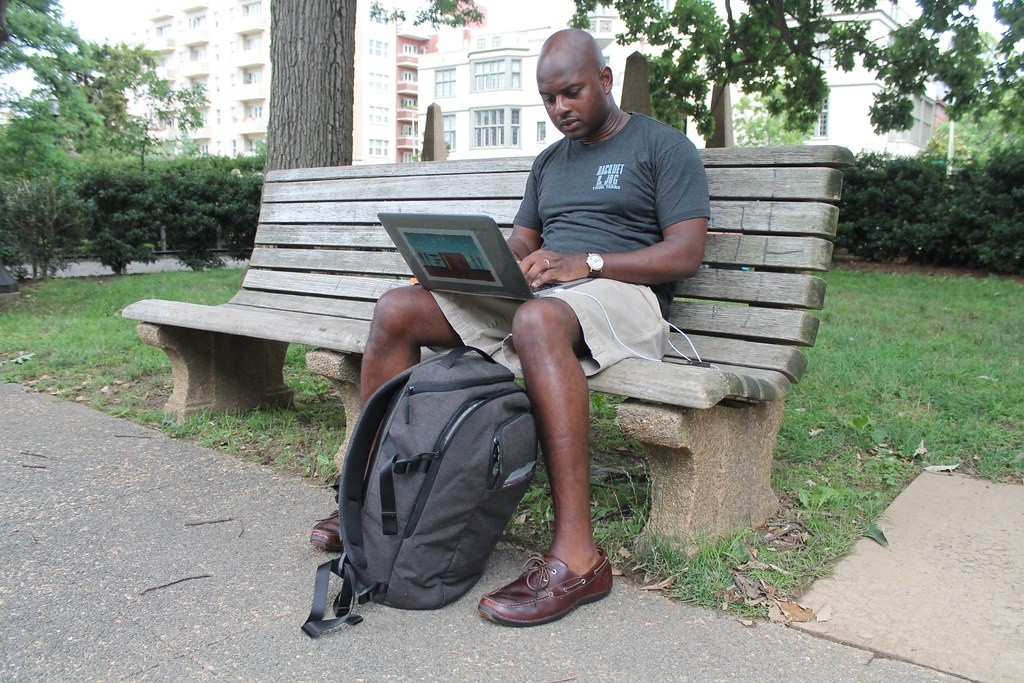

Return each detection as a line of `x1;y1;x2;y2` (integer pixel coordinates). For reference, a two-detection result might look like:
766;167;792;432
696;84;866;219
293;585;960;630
545;260;550;270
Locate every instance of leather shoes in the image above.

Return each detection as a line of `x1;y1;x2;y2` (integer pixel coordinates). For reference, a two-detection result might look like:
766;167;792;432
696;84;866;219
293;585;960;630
478;545;614;628
310;509;342;551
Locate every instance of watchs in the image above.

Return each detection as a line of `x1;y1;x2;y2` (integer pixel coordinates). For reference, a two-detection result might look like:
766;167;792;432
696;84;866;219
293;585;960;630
586;252;604;279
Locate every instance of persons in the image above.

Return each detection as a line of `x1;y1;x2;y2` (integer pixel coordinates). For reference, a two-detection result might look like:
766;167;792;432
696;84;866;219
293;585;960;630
309;28;712;628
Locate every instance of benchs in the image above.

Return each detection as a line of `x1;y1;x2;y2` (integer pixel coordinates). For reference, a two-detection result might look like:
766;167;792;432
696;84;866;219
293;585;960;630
122;143;856;561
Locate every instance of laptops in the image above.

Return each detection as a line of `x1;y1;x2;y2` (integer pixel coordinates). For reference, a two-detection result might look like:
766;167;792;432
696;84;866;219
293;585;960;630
376;213;592;300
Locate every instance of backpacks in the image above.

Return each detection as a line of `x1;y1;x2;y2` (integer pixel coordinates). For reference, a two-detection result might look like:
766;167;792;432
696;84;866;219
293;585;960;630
300;344;539;640
